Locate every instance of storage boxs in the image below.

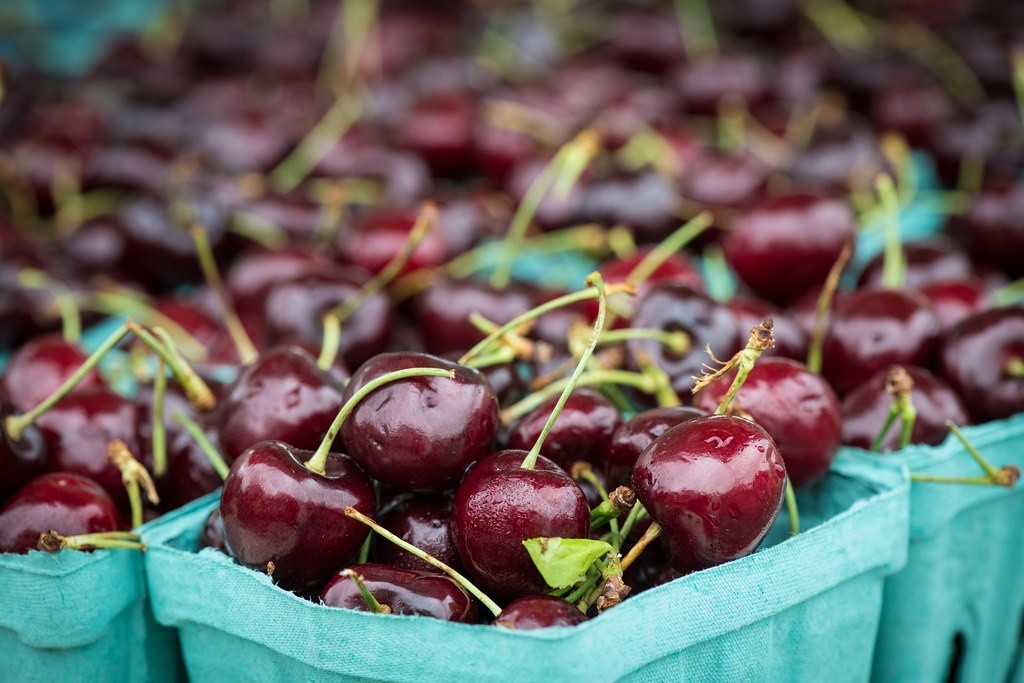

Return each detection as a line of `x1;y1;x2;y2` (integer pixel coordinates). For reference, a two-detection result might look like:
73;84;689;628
0;0;1024;683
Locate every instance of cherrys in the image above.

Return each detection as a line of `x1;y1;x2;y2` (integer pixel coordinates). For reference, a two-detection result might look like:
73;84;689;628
0;0;1024;635
